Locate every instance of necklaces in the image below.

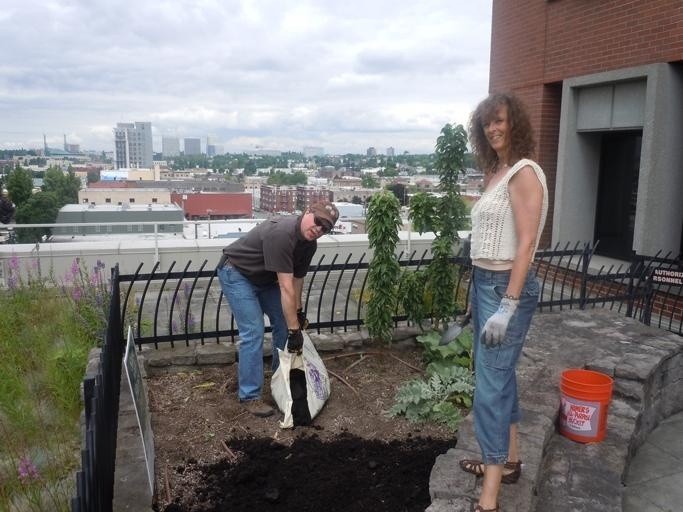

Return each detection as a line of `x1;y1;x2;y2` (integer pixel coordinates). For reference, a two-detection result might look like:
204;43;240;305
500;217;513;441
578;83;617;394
494;161;508;173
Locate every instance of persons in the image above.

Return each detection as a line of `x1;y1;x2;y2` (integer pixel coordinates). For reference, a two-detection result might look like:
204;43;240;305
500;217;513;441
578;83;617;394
215;200;339;417
457;92;549;512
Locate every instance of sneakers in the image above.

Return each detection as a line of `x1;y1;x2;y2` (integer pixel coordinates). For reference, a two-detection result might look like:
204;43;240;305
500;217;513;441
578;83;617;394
242;400;274;417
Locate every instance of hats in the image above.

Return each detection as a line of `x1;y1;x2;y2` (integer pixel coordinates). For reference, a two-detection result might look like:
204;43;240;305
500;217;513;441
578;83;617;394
310;200;339;227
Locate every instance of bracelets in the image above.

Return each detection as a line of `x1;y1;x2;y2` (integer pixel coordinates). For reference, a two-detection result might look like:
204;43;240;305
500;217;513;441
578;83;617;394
502;294;520;302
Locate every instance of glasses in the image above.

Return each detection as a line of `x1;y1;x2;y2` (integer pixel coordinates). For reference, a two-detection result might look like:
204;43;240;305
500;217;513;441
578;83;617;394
314;217;330;233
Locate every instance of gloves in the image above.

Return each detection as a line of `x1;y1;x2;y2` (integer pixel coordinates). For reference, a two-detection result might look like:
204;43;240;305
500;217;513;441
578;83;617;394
480;298;520;349
287;326;303;356
296;308;309;331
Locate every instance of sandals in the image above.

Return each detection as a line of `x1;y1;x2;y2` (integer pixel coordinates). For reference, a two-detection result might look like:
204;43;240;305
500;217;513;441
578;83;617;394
474;502;499;512
460;460;522;484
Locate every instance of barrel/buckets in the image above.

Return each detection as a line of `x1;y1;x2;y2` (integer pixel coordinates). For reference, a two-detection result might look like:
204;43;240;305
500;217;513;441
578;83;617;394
559;369;614;443
